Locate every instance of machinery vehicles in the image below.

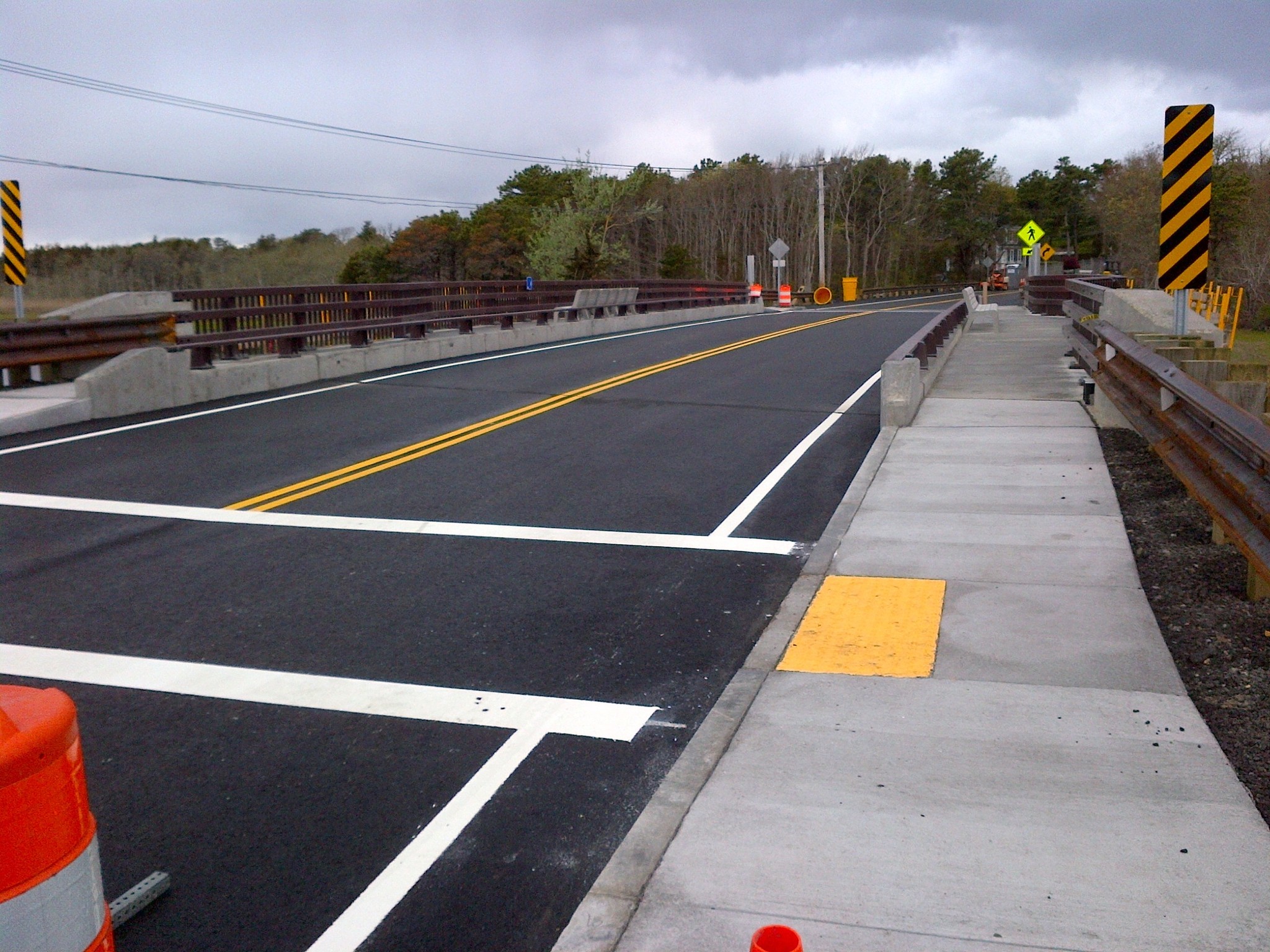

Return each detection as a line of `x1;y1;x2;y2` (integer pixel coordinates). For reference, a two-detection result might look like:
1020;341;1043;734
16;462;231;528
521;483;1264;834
979;263;1009;291
1101;260;1133;289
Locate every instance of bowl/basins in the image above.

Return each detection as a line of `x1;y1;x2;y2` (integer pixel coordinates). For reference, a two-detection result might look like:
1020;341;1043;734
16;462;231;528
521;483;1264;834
813;287;831;305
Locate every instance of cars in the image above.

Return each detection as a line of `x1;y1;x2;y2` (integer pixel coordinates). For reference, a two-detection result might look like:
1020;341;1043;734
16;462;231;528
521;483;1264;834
1077;270;1094;276
1006;264;1020;274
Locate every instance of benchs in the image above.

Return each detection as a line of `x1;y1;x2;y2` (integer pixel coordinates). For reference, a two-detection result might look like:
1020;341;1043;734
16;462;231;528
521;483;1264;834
963;287;998;330
554;287;639;321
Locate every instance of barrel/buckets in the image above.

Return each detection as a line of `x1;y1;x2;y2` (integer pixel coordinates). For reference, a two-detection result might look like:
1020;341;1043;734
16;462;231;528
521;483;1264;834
842;277;858;301
